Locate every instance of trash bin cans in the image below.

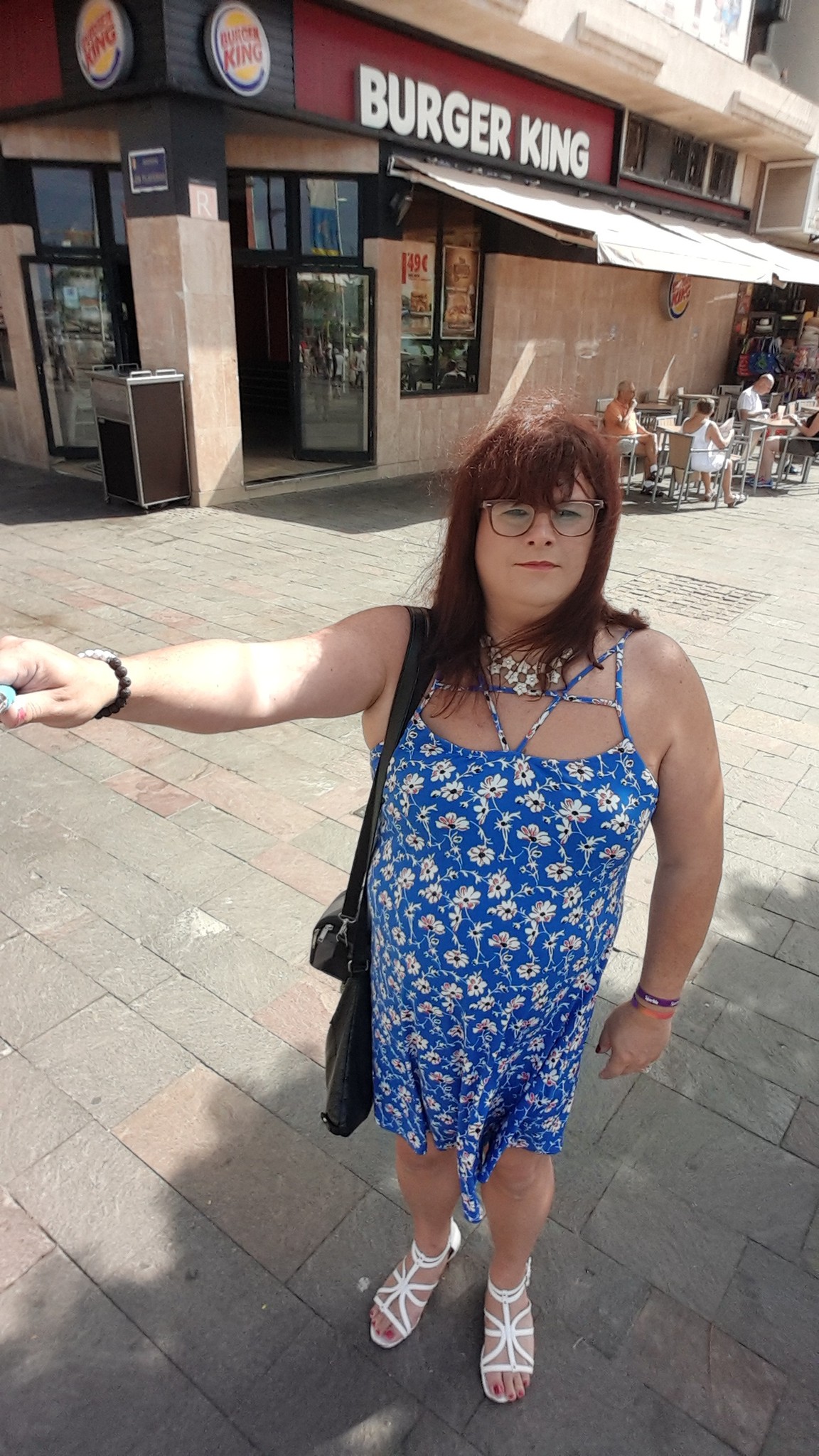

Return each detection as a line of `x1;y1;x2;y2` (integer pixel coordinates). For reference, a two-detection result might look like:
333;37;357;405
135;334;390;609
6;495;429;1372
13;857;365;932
87;362;190;512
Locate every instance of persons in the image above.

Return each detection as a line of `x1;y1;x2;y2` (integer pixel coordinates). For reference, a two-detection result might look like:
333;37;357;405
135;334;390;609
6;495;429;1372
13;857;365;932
406;354;471;390
299;337;367;421
0;395;724;1403
48;326;118;397
603;381;666;498
737;373;802;475
744;383;819;488
679;397;748;508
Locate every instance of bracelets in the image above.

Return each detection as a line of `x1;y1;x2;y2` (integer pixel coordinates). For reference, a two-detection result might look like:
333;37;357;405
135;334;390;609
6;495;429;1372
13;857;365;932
631;994;675;1019
635;984;679;1006
75;649;131;719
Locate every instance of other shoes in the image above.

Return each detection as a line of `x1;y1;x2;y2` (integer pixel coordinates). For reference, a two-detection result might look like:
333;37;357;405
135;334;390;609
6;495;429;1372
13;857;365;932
783;465;801;473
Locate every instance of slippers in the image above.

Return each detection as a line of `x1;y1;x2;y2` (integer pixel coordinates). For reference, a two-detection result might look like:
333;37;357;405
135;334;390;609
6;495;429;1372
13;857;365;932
728;493;748;508
705;492;718;502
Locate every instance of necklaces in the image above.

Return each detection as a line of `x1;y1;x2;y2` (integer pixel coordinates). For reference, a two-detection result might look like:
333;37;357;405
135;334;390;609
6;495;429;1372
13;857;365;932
479;635;573;696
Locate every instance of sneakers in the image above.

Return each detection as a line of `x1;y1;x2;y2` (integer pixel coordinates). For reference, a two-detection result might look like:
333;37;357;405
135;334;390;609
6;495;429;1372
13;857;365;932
745;475;756;483
749;476;772;487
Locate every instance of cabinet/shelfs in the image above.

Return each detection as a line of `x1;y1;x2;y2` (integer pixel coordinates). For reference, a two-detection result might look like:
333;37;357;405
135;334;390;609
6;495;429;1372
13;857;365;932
85;362;192;510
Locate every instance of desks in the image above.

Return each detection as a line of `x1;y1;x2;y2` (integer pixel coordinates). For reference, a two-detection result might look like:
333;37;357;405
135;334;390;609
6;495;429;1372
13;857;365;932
746;417;798;485
656;426;680;497
676;393;719;415
634;403;673;431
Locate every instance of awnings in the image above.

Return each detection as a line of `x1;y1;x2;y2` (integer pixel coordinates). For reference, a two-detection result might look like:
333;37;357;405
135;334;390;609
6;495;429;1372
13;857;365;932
388;154;819;286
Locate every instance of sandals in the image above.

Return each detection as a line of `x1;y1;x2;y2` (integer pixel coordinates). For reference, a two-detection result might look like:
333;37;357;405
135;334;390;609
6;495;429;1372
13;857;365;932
371;1215;462;1349
480;1257;534;1402
641;485;664;496
646;471;663;483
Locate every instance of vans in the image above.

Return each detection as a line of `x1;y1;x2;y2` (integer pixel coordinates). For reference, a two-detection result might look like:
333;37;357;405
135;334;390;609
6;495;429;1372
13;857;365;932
402;336;475;362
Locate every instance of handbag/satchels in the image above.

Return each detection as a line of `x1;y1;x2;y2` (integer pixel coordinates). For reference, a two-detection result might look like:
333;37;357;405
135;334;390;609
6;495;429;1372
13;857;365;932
310;885;374;1136
736;337;785;377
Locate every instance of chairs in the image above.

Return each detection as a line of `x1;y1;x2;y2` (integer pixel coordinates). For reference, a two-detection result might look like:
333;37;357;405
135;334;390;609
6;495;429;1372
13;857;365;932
567;384;819;513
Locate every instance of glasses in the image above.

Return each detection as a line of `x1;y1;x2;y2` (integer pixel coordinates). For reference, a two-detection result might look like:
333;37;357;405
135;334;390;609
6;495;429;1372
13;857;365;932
477;499;604;537
